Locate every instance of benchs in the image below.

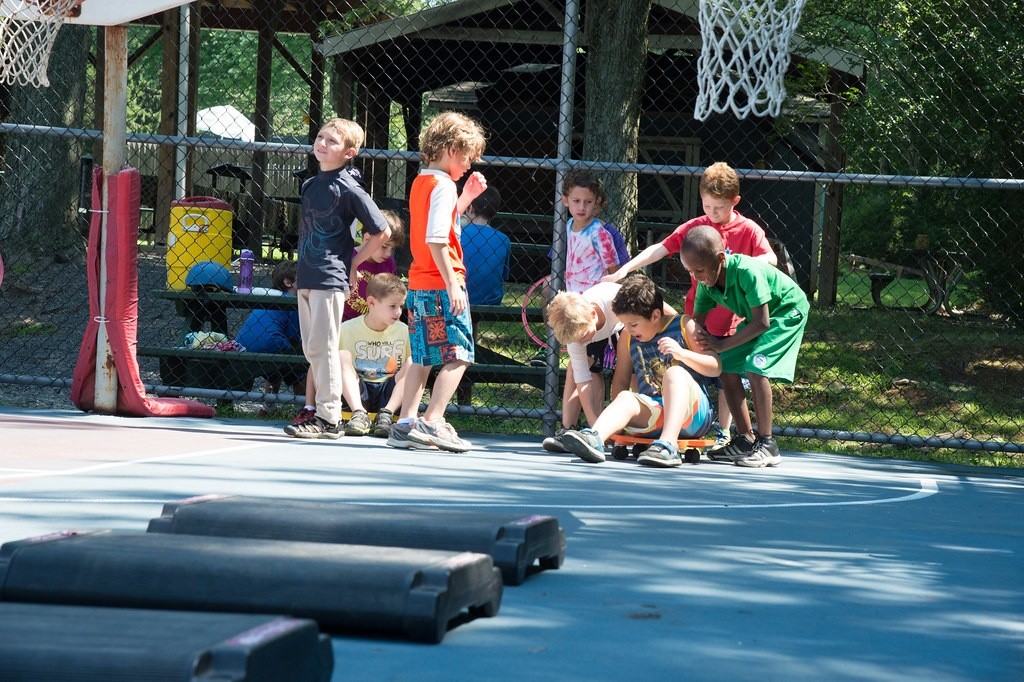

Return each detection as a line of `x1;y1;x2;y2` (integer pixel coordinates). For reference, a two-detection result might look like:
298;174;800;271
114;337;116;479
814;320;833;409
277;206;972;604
868;272;896;312
131;284;616;408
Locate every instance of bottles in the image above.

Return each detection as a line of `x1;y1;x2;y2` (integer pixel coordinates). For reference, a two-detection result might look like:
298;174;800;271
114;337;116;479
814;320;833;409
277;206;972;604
741;378;750;389
179;395;218;408
238;253;252;294
234;403;281;414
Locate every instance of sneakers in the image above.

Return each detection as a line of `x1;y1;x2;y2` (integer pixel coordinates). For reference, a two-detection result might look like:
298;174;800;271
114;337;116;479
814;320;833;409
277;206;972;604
707;431;755;462
712;424;732;455
636;440;682;468
344;409;374;436
373;408;394;437
542;424;584;453
292;408;316;425
734;429;781;467
563;427;606;463
386;420;439;450
407;416;473;453
284;415;345;440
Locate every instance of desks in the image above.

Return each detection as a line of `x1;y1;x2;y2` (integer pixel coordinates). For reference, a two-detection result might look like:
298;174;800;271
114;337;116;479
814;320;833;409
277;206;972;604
894;245;985;321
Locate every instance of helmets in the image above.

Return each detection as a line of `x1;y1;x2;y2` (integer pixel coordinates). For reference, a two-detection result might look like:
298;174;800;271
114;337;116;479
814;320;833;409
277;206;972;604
185;260;233;294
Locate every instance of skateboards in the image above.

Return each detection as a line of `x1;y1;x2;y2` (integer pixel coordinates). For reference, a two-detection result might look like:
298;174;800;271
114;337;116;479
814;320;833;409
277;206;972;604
608;434;715;462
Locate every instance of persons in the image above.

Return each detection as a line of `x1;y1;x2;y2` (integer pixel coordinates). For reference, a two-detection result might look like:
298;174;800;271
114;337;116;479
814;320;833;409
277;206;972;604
680;225;810;468
283;117;392;440
288;210;405;426
543;167;630;453
386;112;485;452
597;162;777;449
235;261;311;395
562;274;721;467
459;186;511;341
338;272;412;437
548;280;623;429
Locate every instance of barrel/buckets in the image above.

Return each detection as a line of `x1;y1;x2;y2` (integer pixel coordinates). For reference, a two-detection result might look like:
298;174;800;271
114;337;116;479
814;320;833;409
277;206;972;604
165;207;234;291
